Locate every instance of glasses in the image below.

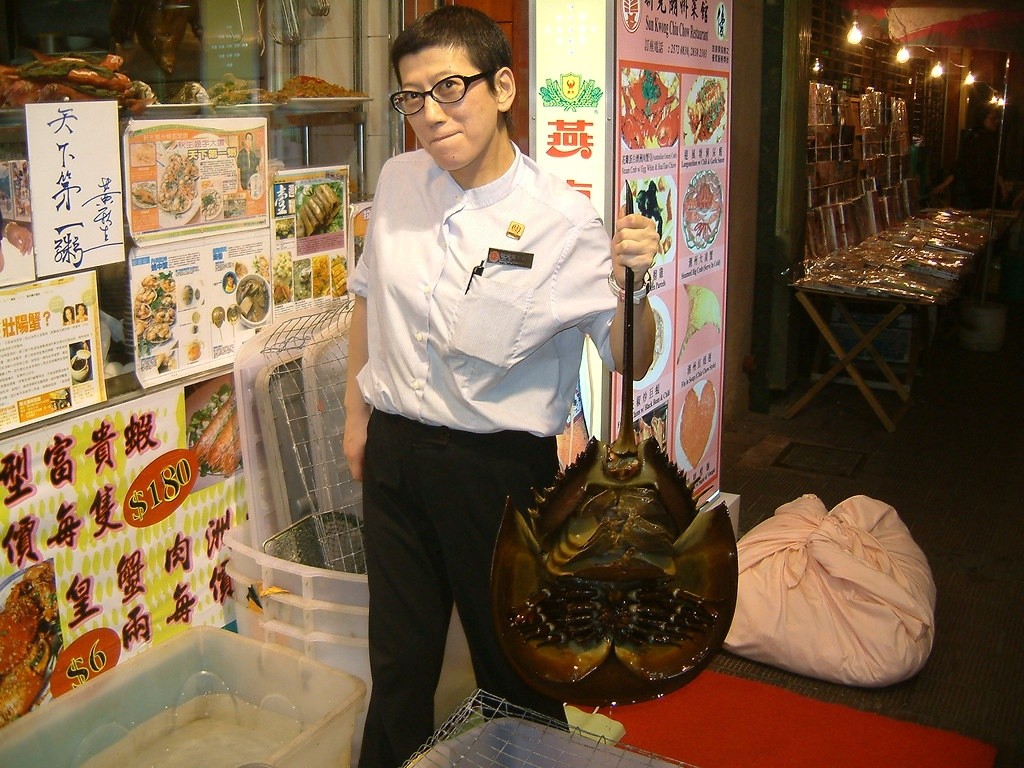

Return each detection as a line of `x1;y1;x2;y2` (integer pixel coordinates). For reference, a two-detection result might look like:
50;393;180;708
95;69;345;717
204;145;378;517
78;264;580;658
986;115;1001;122
388;68;499;116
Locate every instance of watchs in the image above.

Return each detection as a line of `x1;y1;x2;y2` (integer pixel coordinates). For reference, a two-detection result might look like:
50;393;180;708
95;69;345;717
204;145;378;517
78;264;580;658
608;269;652;304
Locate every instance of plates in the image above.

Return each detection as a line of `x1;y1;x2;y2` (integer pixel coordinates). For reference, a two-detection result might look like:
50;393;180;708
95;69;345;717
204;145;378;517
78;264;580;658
675;379;718;472
680;169;722;255
134;269;176;345
185;340;204;364
82;51;109;54
187;391;242;477
620;68;677;150
131;143;156;168
201;186;223;215
131;180;158;209
157;140;202;228
621;175;677;267
684;74;728;147
633;295;672;392
0;558;58;713
2;96;375;122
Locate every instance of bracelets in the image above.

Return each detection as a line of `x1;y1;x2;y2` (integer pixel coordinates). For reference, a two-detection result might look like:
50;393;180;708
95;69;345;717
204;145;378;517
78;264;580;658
4;221;16;238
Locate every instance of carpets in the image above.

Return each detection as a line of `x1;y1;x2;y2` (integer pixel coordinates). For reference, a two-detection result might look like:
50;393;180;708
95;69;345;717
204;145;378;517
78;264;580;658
570;668;998;768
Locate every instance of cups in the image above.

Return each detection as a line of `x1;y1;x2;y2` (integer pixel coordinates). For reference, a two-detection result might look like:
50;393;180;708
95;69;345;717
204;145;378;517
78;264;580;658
69;350;91;383
38;32;61;51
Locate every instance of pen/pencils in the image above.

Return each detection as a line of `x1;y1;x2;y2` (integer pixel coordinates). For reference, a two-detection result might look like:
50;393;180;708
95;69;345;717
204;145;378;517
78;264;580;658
464;260;485;294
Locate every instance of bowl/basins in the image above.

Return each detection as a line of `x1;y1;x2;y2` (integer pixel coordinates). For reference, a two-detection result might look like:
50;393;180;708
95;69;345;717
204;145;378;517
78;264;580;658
66;37;92;50
234;273;272;328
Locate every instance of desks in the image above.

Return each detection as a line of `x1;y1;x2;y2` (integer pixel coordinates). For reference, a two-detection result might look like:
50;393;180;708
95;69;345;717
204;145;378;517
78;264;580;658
785;206;1022;432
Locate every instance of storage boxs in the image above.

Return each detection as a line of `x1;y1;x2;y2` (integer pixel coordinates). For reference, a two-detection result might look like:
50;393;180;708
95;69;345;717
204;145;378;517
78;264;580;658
414;717;685;768
0;624;367;768
224;310;467;768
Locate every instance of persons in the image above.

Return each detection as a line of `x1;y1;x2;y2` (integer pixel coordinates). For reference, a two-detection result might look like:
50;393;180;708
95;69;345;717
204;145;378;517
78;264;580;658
0;209;33;273
62;303;88;327
342;5;661;768
238;133;260;191
932;99;1006;209
